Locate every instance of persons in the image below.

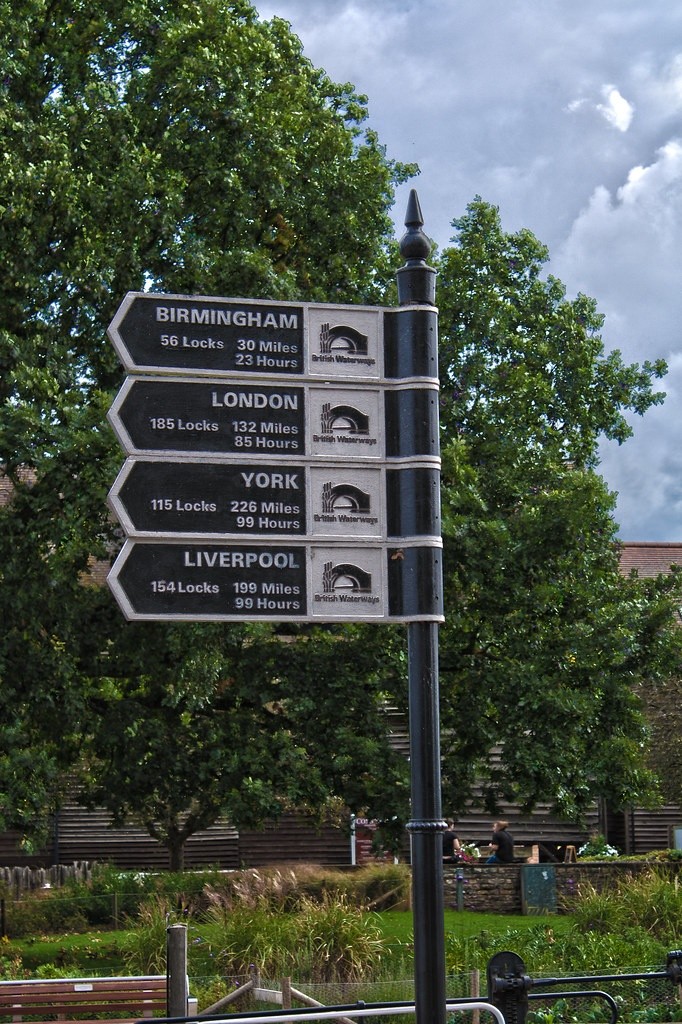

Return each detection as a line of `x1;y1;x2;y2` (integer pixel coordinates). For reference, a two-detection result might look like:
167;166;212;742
442;819;460;864
486;821;514;863
